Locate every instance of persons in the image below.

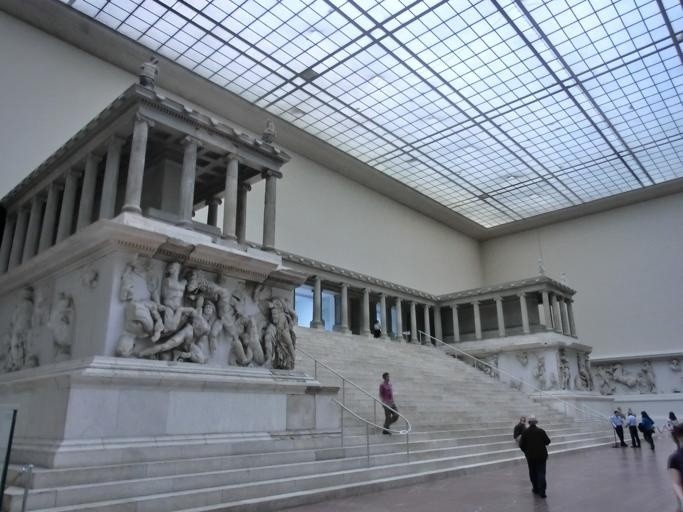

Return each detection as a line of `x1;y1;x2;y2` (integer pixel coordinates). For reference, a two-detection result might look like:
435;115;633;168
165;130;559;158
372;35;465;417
609;406;654;451
666;423;682;512
373;319;381;338
0;251;298;375
665;412;680;449
516;348;682;394
378;371;399;434
512;417;526;443
518;415;550;498
